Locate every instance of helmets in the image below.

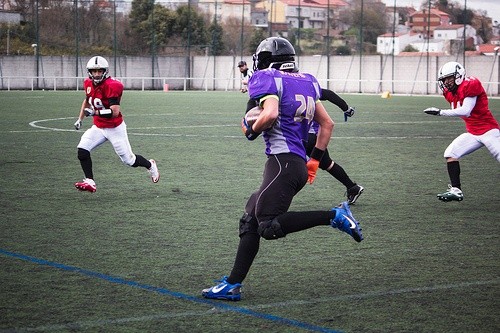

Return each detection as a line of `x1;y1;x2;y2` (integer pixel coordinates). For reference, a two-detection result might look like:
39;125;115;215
438;62;466;93
86;55;109;83
252;36;295;72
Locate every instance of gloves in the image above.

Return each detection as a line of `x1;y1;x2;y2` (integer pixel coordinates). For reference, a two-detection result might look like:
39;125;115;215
74;120;81;130
84;106;95;116
241;116;249;135
306;157;320;183
424;107;440;116
344;107;354;121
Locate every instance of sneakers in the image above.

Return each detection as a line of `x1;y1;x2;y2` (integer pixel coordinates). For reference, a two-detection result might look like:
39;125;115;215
340;184;363;205
437;184;464;202
202;277;242;301
329;201;363;242
147;159;160;183
75;179;97;192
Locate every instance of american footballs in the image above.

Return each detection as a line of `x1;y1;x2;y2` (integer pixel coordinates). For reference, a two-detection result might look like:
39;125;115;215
244;107;265;127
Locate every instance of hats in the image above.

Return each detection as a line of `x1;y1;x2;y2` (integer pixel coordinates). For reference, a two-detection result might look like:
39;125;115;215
237;61;246;67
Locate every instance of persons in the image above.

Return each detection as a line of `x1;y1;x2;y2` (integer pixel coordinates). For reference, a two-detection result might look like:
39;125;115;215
238;61;258;93
74;56;160;193
246;89;365;205
423;62;500;203
202;36;363;300
